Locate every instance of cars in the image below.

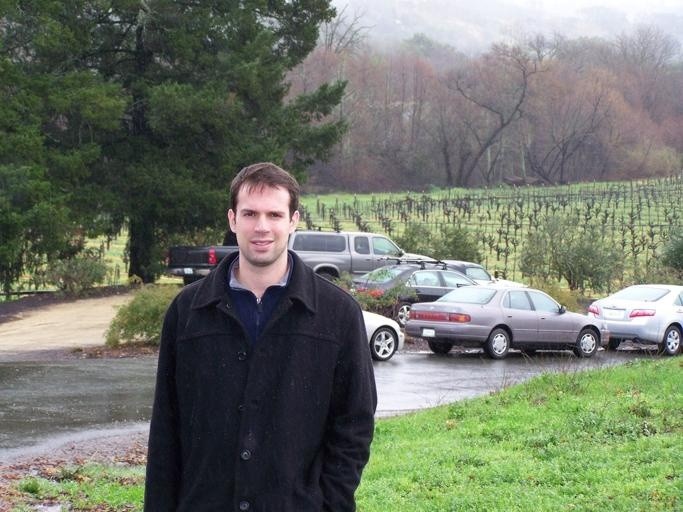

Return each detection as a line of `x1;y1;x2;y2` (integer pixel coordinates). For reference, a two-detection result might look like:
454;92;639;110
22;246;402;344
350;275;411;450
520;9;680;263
349;257;482;330
437;259;528;288
362;311;407;362
587;284;683;356
405;286;611;360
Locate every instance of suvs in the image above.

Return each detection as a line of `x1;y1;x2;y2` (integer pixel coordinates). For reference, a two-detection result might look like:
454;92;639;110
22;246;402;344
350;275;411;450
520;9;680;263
286;230;438;291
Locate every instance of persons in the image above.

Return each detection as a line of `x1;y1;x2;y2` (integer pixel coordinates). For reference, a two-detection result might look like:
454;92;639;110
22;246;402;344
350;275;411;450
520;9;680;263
142;162;378;511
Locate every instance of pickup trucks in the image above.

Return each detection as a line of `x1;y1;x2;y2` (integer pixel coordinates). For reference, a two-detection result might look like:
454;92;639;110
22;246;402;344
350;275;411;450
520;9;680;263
165;230;239;286
